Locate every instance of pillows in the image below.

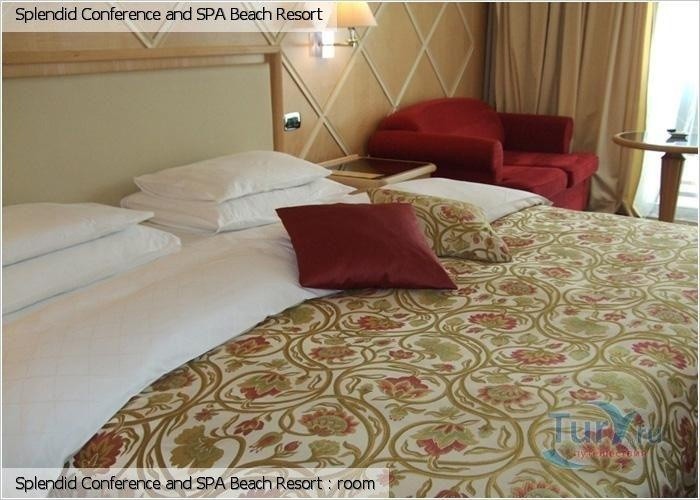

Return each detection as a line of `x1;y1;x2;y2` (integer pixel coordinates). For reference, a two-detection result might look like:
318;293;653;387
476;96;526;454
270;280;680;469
367;188;513;263
133;150;333;205
274;202;459;289
2;224;182;316
120;178;358;236
2;201;154;267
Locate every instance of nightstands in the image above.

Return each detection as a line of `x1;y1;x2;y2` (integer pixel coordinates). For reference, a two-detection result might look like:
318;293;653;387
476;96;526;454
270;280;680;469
315;153;437;185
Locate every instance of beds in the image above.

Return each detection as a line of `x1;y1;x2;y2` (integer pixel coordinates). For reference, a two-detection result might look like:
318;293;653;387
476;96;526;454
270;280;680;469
2;46;698;498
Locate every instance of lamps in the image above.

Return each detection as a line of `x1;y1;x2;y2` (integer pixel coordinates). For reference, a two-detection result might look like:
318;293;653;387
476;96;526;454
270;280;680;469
308;1;378;59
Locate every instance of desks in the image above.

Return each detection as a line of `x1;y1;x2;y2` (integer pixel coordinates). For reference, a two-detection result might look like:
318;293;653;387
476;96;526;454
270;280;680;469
613;129;698;222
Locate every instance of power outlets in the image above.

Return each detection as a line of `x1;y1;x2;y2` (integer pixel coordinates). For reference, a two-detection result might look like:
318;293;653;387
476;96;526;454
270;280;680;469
283;112;301;131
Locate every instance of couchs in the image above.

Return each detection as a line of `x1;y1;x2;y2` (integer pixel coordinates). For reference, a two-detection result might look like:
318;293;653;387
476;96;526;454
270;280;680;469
368;97;599;210
